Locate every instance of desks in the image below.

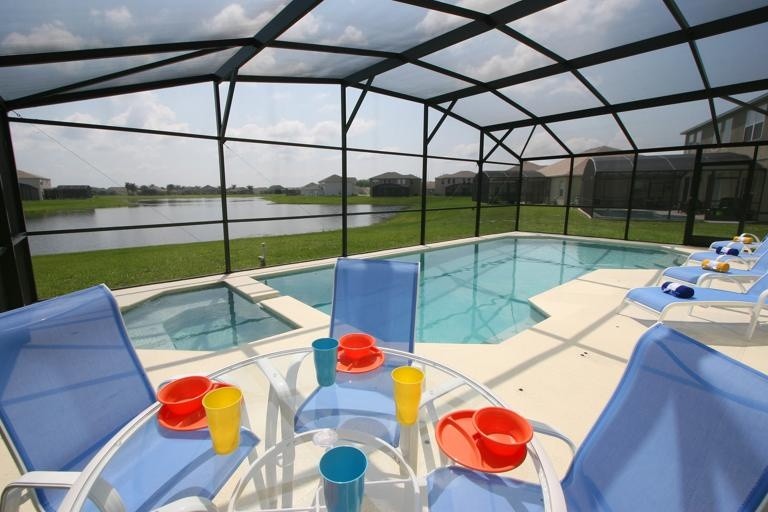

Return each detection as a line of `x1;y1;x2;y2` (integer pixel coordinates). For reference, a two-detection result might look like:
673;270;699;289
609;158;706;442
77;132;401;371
55;346;566;511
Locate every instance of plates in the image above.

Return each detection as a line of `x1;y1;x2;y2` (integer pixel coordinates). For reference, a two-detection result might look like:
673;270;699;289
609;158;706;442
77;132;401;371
156;382;244;431
435;409;528;473
336;345;385;374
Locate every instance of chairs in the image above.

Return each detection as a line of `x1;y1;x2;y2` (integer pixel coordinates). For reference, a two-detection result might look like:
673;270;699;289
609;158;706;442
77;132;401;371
428;322;767;511
0;283;268;511
280;258;421;510
616;233;768;341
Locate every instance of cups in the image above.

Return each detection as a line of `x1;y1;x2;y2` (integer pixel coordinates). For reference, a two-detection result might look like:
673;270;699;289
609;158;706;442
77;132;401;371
317;445;368;511
311;337;339;386
392;366;426;426
201;386;243;455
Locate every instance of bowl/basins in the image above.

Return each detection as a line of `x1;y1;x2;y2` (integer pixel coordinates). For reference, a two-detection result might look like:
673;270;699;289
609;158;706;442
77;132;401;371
473;406;534;457
338;333;376;361
158;376;213;416
